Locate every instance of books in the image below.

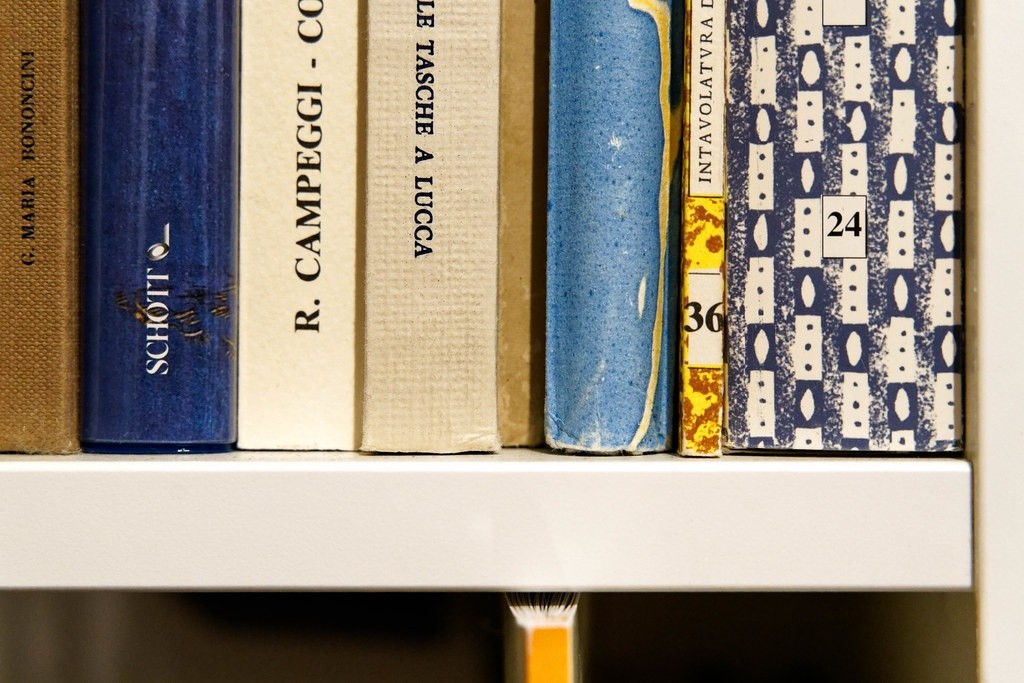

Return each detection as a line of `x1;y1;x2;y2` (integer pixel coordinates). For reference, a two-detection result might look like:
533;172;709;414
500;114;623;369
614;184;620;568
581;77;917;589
505;592;590;683
0;0;965;460
